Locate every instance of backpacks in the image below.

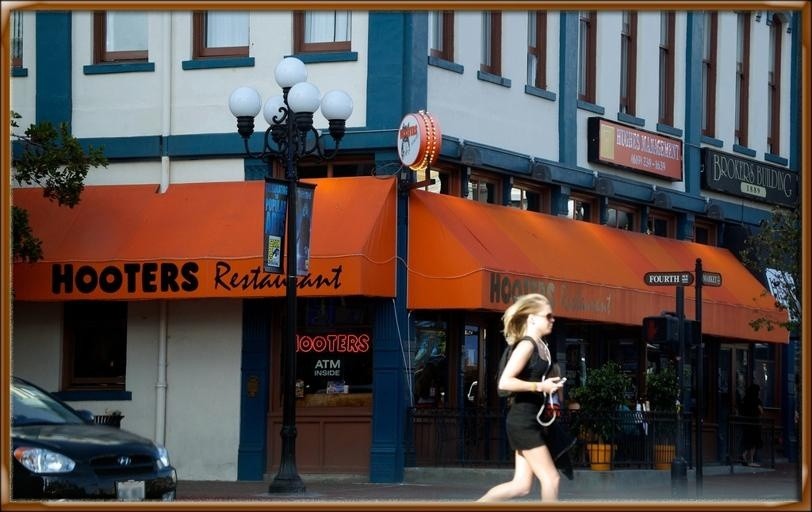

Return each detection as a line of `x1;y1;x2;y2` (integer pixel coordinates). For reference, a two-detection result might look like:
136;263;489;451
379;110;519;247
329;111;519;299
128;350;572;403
496;336;540;398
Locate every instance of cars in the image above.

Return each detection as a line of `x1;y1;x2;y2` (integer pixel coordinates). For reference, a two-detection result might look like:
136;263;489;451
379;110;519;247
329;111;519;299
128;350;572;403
11;375;180;506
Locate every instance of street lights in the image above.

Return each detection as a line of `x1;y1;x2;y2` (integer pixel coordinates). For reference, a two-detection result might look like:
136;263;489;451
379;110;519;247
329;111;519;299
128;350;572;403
230;55;358;497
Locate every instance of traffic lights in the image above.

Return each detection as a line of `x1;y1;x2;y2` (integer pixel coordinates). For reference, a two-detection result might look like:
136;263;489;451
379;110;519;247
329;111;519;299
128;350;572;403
685;319;702;350
644;317;678;347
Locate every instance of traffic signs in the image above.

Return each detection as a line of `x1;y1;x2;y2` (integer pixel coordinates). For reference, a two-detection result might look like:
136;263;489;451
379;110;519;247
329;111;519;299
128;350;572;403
703;270;723;287
644;272;694;286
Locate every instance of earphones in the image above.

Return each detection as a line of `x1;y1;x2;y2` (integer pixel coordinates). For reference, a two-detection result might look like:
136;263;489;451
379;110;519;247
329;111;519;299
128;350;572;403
531;318;533;323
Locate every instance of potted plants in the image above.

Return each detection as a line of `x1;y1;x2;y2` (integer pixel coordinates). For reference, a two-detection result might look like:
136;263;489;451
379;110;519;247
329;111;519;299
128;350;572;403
646;367;684;470
567;360;634;471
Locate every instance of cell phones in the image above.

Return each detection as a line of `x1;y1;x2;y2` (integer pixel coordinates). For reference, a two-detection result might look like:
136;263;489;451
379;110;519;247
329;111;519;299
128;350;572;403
556;377;567;384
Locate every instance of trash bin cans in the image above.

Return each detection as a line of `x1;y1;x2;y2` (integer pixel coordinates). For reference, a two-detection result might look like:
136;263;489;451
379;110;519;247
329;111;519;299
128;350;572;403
95;415;124;428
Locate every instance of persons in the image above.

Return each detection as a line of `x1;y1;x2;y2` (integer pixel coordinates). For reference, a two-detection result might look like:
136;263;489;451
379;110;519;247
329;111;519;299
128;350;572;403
741;374;763;467
475;292;568;505
538;358;574;481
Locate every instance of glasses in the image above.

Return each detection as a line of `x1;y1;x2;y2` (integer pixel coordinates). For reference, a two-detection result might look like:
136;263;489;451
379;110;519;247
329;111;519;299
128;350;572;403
538;313;555;322
